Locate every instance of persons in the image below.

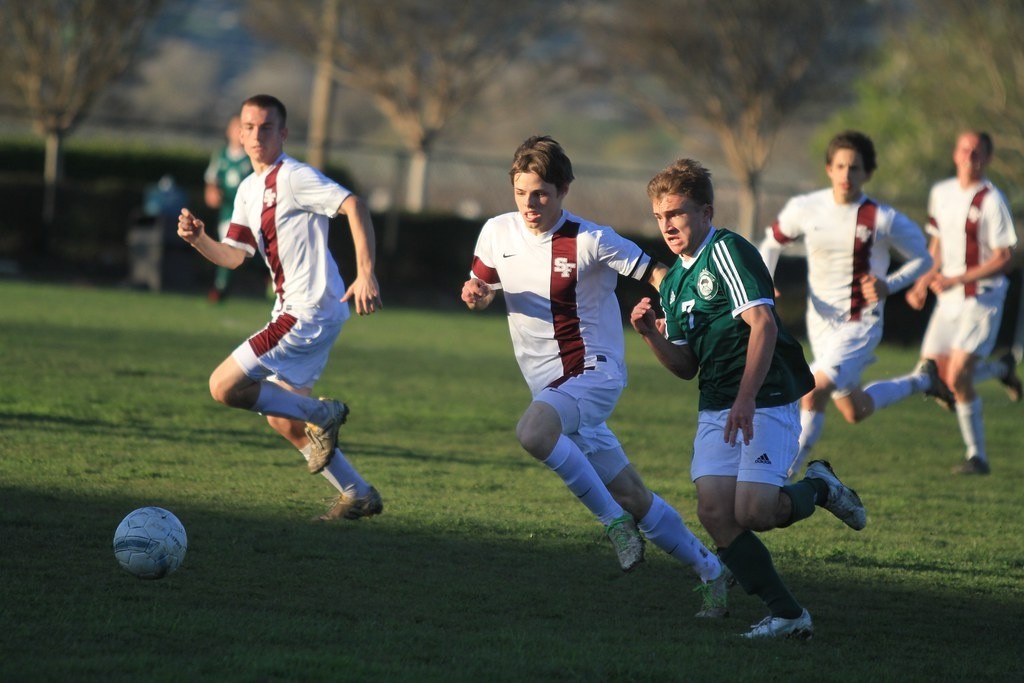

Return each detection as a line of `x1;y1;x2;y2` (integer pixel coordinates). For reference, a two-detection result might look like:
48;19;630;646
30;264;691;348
904;128;1024;477
757;131;959;489
201;113;271;300
629;158;867;645
177;93;384;520
461;134;737;618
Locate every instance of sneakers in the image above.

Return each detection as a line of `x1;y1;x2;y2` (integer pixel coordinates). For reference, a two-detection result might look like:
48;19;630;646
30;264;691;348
604;508;647;571
307;395;349;476
916;359;953;411
803;459;869;531
998;353;1022;402
324;487;383;521
691;560;736;618
742;608;813;637
951;456;989;477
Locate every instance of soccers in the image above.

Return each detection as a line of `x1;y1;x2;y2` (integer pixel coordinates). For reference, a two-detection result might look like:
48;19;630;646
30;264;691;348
112;505;189;580
